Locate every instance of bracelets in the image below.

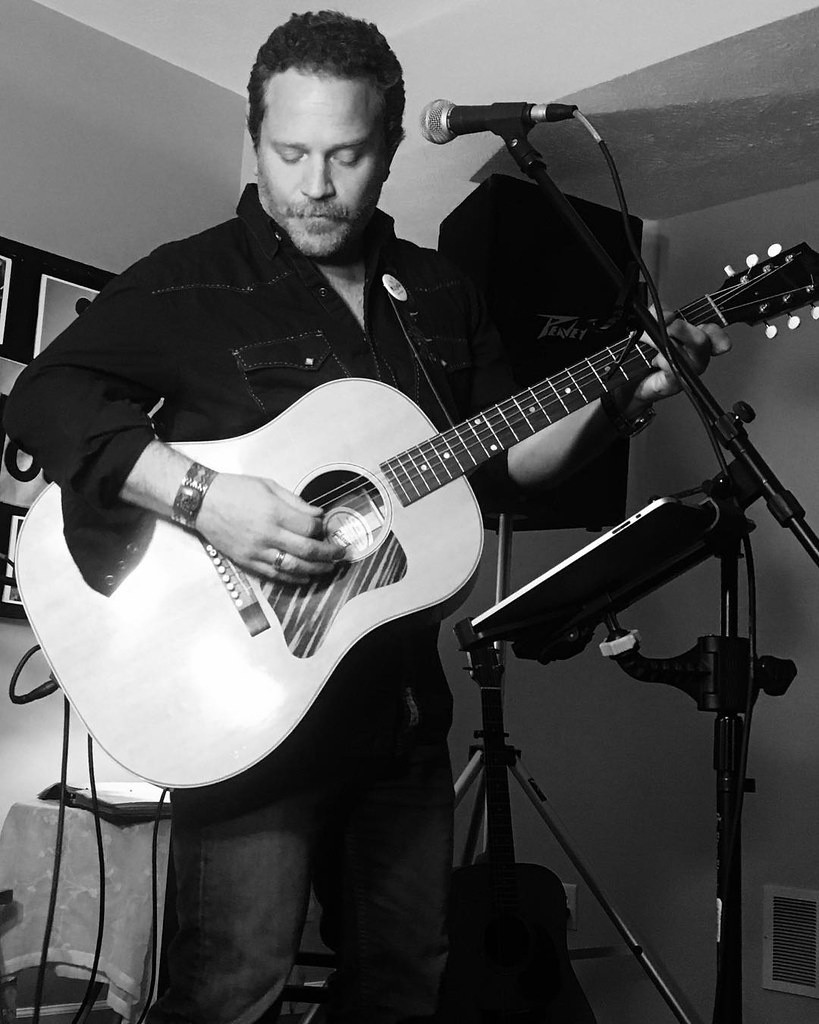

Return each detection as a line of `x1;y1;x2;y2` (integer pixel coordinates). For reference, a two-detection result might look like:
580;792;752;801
173;461;218;529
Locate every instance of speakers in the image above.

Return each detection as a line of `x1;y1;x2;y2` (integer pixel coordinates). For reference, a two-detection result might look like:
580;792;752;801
439;175;644;536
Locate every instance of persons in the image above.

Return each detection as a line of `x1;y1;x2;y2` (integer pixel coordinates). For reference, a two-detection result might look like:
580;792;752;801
3;9;731;1024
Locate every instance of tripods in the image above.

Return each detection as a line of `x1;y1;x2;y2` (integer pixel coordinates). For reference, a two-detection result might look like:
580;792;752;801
452;517;689;1024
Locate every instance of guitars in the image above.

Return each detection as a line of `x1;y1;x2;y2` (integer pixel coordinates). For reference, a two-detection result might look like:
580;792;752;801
5;233;819;806
432;615;616;1024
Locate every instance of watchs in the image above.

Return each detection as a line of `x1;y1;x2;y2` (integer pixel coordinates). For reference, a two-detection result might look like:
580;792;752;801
601;389;657;438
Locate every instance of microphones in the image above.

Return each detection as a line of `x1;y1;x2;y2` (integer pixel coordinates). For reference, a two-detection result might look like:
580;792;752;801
418;98;578;144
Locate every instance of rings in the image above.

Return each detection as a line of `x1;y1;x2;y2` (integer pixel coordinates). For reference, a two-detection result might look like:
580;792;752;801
273;549;285;572
